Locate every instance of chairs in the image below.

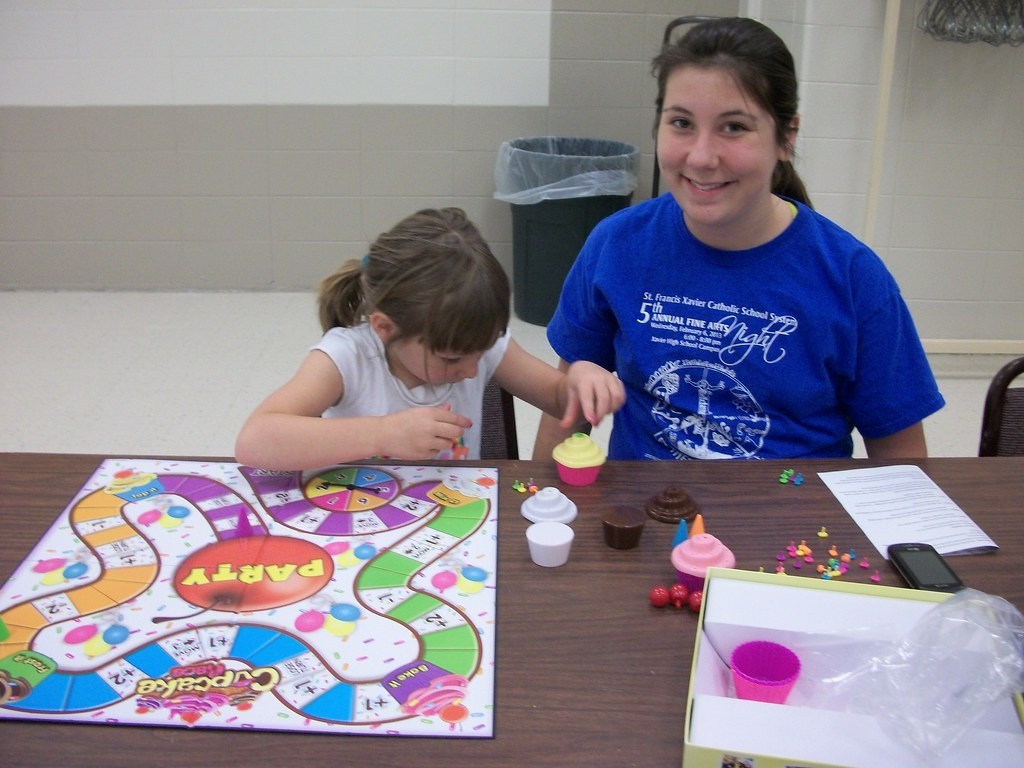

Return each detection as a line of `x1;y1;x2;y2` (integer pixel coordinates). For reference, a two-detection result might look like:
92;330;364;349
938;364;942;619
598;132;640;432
978;357;1024;457
480;381;519;459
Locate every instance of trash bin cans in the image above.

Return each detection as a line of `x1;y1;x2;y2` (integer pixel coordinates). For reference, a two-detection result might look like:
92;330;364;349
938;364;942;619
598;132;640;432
506;133;641;328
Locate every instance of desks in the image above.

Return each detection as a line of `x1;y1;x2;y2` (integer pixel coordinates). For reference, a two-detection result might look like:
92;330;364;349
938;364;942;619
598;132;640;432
0;450;1023;768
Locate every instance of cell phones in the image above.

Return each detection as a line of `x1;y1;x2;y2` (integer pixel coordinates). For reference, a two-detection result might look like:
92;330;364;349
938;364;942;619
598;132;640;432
887;543;967;594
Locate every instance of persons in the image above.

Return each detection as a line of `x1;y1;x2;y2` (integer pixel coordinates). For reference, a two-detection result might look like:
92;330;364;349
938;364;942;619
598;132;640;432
532;16;946;464
235;205;625;472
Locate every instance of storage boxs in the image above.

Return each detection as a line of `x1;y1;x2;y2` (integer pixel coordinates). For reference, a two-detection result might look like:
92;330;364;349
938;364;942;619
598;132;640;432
682;565;1024;768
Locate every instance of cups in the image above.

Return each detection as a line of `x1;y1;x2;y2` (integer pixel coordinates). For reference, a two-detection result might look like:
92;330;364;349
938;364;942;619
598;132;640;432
731;641;801;704
553;457;601;485
525;521;575;568
671;564;704;595
